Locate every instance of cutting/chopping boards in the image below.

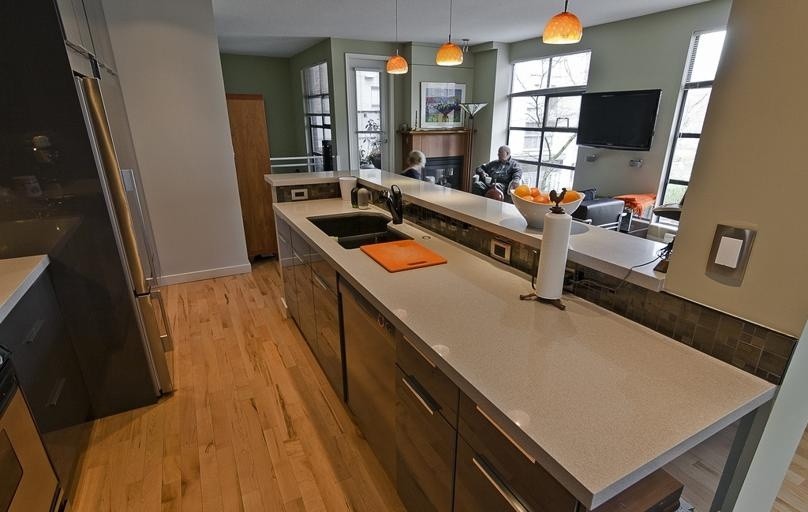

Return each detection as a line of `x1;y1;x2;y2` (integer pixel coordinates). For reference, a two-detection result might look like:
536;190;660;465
359;240;448;273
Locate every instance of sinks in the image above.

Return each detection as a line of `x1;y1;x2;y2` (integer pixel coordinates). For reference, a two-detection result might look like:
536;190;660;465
329;227;413;249
306;212;394;237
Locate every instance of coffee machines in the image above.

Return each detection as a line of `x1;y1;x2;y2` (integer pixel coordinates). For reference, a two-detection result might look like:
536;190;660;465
322;140;333;160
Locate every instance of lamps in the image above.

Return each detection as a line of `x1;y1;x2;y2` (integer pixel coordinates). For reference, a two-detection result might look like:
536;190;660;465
387;1;409;75
435;1;465;67
542;0;584;45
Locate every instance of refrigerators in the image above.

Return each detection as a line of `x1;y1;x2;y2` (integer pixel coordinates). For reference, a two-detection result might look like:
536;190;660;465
0;0;176;434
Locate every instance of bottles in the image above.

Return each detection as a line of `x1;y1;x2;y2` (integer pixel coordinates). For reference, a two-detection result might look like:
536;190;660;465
399;120;408;133
350;186;372;210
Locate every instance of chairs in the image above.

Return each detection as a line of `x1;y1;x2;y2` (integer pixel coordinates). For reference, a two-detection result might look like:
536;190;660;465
472;161;522;203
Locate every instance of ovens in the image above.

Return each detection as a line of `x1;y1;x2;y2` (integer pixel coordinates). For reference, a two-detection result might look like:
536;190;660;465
1;347;72;512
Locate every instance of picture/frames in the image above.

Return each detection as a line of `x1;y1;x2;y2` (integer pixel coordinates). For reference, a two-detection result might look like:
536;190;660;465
419;80;467;128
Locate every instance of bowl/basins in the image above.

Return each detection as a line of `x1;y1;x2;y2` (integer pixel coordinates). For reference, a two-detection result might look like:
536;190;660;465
509;189;586;230
11;176;42;197
369;154;381;169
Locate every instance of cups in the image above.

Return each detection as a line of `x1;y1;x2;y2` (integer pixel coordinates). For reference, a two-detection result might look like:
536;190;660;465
340;177;357;201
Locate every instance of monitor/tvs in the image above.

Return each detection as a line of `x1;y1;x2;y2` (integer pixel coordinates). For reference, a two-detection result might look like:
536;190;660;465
576;89;663;152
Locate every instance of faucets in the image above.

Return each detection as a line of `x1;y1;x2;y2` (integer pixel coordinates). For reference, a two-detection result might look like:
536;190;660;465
380;185;403;225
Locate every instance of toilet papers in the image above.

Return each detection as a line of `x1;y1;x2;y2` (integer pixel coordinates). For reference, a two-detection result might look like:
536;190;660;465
534;208;572;304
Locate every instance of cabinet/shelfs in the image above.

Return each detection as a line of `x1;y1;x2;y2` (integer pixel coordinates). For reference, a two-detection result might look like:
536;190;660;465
2;254;98;495
273;212;346;407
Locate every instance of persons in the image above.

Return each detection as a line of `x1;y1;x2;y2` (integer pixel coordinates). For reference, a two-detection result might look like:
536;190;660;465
474;144;523;202
400;150;427;181
